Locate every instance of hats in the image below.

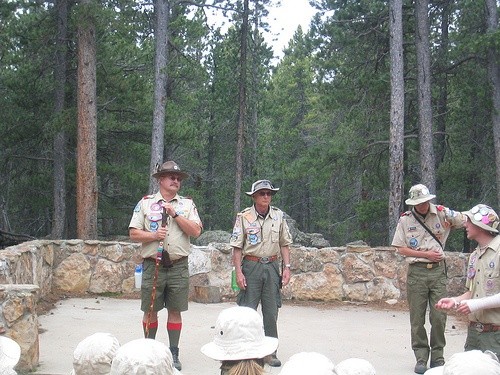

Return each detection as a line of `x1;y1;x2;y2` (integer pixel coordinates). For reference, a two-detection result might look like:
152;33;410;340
279;352;336;375
201;306;279;360
244;180;280;197
334;358;376;375
405;184;436;206
424;350;500;375
109;339;182;375
72;334;121;375
0;336;21;375
151;161;189;179
462;204;500;233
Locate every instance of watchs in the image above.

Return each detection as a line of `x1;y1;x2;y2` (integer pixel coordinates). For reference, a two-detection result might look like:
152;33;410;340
173;212;179;218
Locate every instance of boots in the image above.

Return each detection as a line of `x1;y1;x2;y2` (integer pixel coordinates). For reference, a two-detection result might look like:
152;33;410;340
170;347;181;370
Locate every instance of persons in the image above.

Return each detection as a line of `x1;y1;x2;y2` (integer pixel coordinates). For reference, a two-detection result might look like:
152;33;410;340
0;336;21;375
129;161;202;370
280;352;375;375
435;204;500;361
391;184;467;374
71;332;181;375
424;350;500;375
231;180;292;368
201;307;279;375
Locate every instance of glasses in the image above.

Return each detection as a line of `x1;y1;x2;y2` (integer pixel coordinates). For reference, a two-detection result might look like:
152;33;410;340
161;175;181;181
256;192;271;196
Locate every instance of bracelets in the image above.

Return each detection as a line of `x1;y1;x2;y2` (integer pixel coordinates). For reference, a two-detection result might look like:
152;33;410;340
453;299;459;309
285;265;290;267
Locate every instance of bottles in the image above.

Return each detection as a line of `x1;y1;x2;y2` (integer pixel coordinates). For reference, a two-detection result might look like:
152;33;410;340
134;266;142;289
231;267;240;291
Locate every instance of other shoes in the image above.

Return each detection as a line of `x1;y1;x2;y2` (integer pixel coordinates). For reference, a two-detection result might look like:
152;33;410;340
264;355;281;367
430;363;443;368
415;360;427;374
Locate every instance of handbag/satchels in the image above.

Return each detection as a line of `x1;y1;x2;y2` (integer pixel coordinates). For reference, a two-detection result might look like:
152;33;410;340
231;266;243;297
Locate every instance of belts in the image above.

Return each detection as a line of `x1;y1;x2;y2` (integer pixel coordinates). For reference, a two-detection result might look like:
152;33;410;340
413;262;441;269
146;256;187;264
470;322;500;333
245;255;277;264
